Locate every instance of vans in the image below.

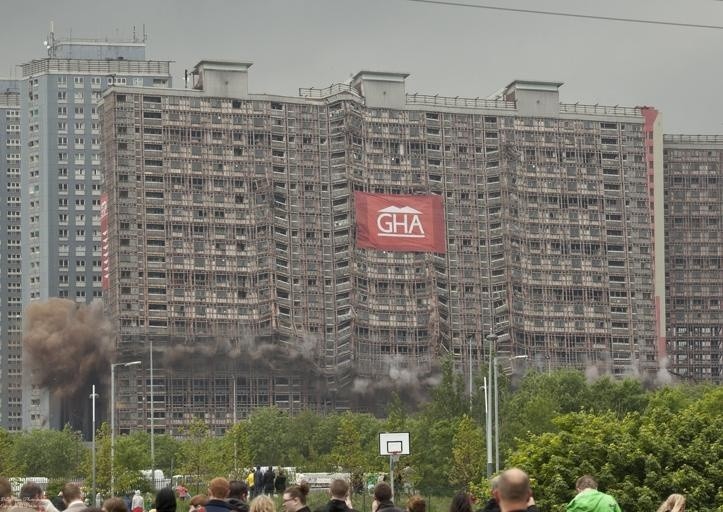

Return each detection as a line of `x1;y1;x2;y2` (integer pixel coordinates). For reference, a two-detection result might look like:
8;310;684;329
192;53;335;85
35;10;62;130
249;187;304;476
136;469;211;492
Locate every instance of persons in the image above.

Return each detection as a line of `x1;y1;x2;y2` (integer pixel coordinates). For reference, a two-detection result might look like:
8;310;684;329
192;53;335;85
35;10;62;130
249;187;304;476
0;466;178;511
658;493;686;512
190;466;427;512
450;468;622;512
175;485;191;501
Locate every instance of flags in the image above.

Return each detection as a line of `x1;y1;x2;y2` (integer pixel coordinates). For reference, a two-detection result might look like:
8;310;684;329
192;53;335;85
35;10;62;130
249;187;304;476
355;192;447;252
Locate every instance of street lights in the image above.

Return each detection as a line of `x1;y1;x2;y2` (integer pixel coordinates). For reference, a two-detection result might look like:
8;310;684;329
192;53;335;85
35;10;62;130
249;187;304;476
478;375;487;434
89;384;99;497
230;372;237;470
492;354;527;485
110;360;141;497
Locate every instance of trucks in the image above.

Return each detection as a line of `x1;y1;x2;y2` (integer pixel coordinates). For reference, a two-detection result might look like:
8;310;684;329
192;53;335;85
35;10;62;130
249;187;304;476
7;477;52;498
